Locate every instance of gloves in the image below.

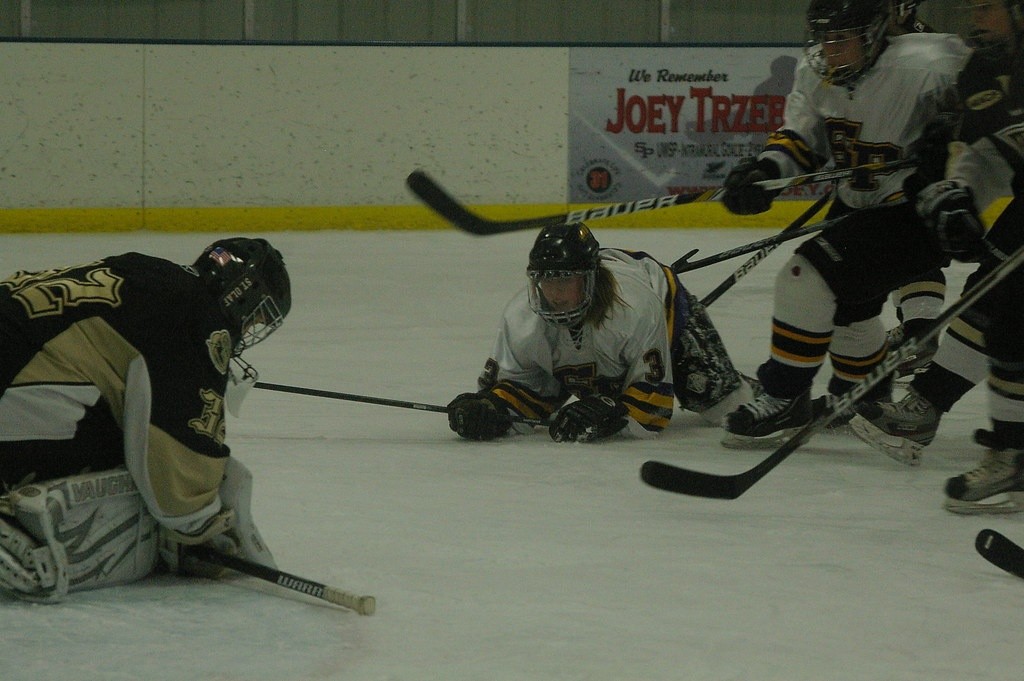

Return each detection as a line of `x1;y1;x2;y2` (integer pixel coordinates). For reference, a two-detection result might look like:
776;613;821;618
446;391;513;443
917;181;985;254
161;511;241;579
722;157;781;215
548;393;629;445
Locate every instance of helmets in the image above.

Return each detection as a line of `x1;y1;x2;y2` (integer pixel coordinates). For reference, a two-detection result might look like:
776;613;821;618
525;223;599;329
955;0;1024;71
193;237;293;355
892;0;921;31
803;0;894;90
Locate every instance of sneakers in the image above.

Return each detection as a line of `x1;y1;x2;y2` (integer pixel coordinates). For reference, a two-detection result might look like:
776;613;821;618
942;448;1024;516
848;392;943;469
886;321;939;389
721;383;812;450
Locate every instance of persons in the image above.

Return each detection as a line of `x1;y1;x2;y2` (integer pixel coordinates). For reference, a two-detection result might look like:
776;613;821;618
851;1;1023;502
719;0;1003;435
447;220;753;440
0;236;291;594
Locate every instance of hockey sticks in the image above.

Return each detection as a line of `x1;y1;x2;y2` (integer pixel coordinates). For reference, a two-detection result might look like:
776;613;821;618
252;381;552;427
406;147;923;235
179;544;376;615
697;190;834;310
668;197;921;273
639;242;1024;502
